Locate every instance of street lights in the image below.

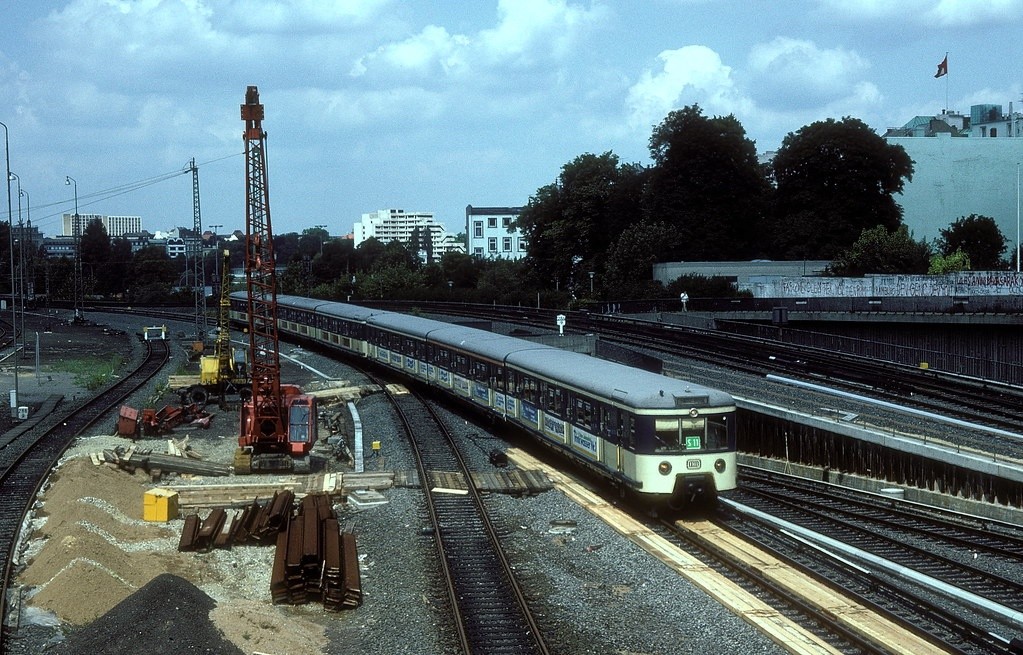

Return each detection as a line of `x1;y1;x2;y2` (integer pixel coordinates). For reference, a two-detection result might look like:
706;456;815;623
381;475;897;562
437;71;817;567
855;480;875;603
66;176;80;308
19;188;30;240
9;172;28;359
589;272;596;297
448;280;453;293
208;224;223;237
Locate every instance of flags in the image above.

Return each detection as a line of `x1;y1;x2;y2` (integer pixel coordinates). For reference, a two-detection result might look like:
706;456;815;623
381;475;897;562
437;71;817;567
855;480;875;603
934;55;947;78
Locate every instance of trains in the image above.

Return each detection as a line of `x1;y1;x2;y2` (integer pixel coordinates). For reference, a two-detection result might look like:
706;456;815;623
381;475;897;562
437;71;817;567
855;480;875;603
227;291;740;515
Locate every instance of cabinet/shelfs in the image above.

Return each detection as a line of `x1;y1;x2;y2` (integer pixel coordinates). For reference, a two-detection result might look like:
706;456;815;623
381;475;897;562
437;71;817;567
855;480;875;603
119;405;138;435
143;488;178;523
